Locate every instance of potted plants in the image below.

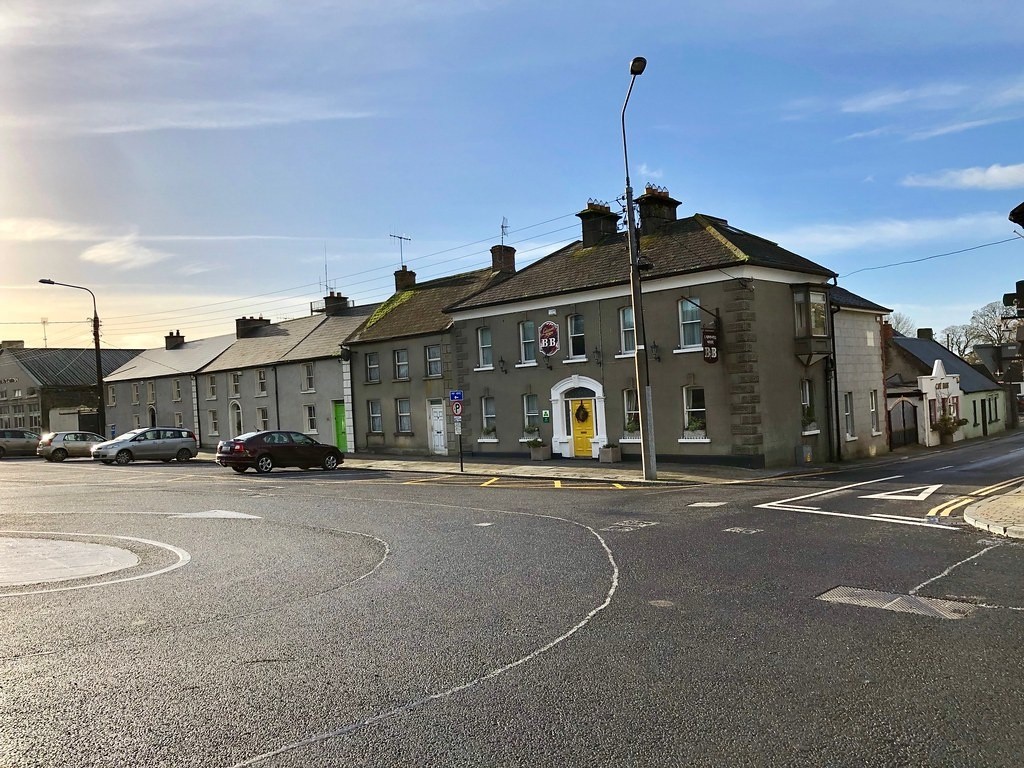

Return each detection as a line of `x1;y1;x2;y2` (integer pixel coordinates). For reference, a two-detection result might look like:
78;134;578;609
802;405;816;431
930;415;967;445
481;426;496;439
524;425;539;438
599;442;621;463
624;417;640;439
527;439;551;461
684;416;706;439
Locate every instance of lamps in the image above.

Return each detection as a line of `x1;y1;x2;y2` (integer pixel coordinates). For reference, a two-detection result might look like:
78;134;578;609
593;346;601;367
650;341;660;362
543;351;552;370
498;356;507;374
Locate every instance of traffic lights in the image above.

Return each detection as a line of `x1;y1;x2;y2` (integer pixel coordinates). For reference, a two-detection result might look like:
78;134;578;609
1002;280;1024;318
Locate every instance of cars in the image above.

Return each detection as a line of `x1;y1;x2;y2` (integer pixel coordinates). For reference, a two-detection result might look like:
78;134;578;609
91;426;199;466
0;429;45;460
36;431;109;462
215;424;344;475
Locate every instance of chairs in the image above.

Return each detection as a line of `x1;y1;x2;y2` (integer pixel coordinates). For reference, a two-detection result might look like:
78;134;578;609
266;436;276;443
278;436;285;443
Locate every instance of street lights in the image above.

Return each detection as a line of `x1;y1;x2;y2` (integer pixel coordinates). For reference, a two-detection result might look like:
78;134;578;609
617;55;660;479
39;277;105;438
